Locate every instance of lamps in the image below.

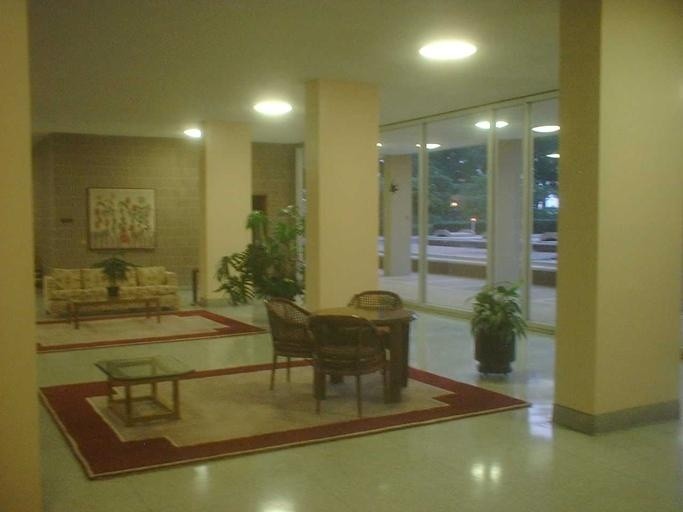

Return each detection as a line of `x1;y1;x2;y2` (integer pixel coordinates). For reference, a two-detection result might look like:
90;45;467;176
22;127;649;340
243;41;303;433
389;176;400;192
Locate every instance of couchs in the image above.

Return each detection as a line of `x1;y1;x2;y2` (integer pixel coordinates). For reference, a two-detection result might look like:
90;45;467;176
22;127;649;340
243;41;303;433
42;271;181;319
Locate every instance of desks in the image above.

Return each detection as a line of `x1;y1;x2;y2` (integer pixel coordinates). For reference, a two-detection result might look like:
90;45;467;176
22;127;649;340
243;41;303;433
312;307;413;403
94;354;194;427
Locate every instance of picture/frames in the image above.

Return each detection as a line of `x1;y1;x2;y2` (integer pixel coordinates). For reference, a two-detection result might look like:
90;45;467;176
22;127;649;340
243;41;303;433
87;187;158;251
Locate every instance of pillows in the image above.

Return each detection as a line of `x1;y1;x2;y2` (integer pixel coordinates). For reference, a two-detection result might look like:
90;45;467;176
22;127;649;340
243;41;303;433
52;266;167;289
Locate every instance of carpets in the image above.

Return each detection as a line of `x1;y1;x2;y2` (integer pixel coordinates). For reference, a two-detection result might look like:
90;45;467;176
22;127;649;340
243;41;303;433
37;360;531;481
36;310;265;353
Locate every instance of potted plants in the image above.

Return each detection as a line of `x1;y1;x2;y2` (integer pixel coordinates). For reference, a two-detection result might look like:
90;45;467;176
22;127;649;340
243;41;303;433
464;279;528;373
104;258;131;296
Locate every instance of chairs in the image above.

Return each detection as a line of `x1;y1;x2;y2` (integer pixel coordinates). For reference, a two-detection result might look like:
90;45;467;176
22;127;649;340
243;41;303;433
264;297;319;398
349;292;403;310
306;313;388;416
